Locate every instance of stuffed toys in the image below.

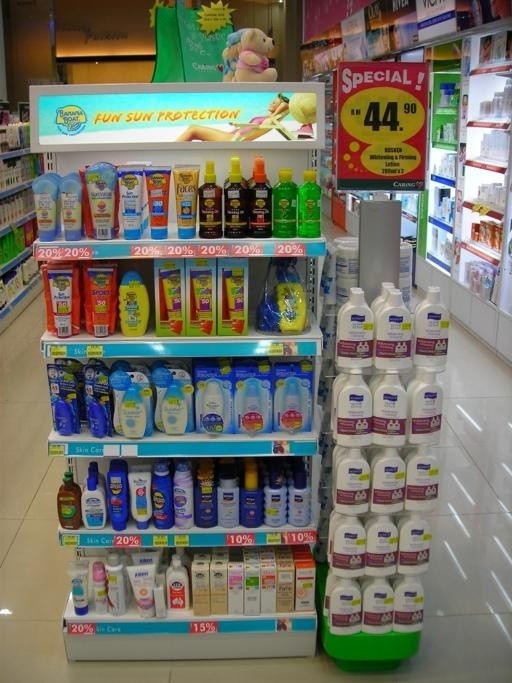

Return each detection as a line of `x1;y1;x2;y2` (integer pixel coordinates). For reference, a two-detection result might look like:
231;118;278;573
220;26;280;82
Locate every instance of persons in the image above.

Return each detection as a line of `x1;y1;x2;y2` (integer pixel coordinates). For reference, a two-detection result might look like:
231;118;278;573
179;91;290;142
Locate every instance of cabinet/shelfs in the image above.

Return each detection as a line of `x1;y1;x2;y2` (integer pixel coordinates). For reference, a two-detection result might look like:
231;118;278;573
1;97;47;325
27;147;322;668
302;14;512;370
